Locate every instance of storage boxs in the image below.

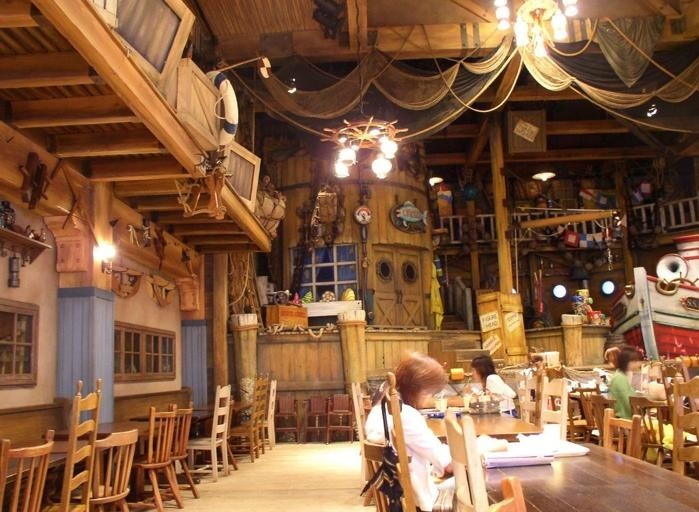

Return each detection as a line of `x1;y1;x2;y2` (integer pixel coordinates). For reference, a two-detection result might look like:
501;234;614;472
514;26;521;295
265;304;309;331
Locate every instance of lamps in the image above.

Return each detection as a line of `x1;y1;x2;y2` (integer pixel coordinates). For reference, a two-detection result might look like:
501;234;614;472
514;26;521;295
319;115;409;181
532;171;556;185
429;177;443;188
493;0;579;58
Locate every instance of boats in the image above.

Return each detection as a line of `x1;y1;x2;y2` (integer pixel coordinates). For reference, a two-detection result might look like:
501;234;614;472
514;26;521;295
602;229;699;378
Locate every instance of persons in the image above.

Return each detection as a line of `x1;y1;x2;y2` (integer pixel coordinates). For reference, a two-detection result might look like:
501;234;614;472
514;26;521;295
530;358;549;400
609;346;686;464
464;355;518;417
363;356;456;512
604;346;620;368
531;355;544;371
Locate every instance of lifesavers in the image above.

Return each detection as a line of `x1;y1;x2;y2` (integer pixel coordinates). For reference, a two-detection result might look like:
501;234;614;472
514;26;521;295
205;71;239;145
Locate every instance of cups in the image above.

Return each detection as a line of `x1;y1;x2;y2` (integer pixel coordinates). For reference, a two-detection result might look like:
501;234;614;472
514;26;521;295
435;392;500;414
587;310;602;325
264;291;277;305
